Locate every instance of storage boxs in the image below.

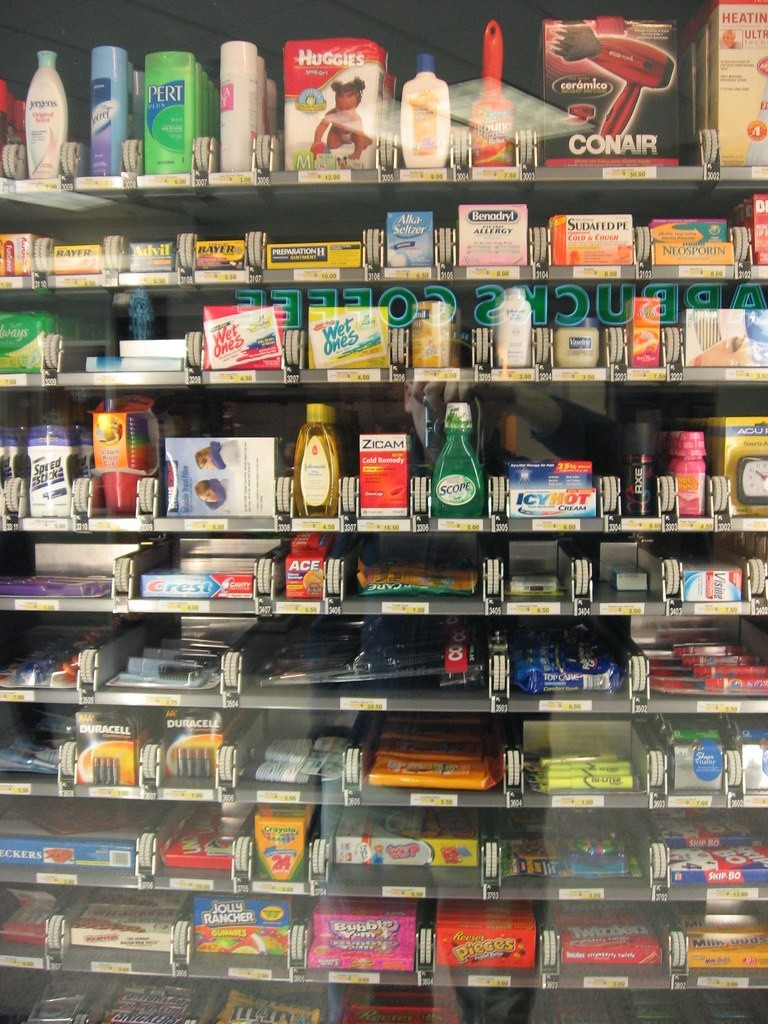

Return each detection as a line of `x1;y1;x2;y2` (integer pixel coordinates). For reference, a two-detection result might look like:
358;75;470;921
534;17;677;166
163;435;276;518
70;906;179;952
681;308;768;367
334;807;478;868
678;0;767;166
0;794;171;870
667;846;767;883
657;820;767;847
90;887;190;912
645;807;768;821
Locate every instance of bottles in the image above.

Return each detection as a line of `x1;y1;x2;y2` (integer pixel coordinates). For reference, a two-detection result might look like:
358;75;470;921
432;400;485;518
399;53;452;168
293;403;339;517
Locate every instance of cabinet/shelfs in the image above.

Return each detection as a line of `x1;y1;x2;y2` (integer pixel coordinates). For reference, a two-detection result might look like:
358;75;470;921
0;0;767;1024
